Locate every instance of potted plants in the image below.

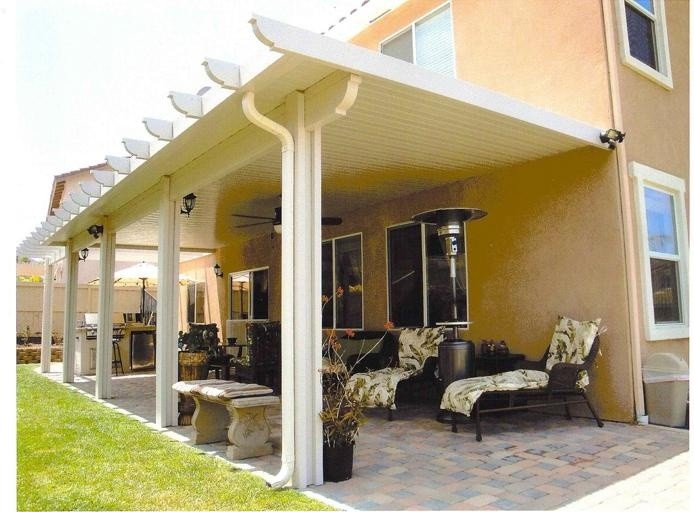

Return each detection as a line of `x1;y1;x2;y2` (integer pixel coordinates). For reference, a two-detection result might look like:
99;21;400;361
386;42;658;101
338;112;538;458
322;284;393;484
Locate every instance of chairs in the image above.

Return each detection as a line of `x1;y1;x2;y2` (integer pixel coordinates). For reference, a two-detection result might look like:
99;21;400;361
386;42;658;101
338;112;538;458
225;318;281;395
177;322;221;379
350;318;444;432
440;316;611;444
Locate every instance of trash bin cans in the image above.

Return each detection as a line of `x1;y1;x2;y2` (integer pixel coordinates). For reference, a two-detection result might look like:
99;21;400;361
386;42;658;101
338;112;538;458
642;352;689;428
438;339;475;398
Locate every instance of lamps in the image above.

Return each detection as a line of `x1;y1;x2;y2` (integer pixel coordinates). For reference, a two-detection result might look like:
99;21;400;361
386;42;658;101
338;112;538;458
413;207;487;423
272;223;284;235
213;261;226;279
79;248;89;262
180;192;199;218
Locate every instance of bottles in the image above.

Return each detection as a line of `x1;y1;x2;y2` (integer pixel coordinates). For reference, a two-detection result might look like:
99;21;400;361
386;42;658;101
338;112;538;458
480;339;508;355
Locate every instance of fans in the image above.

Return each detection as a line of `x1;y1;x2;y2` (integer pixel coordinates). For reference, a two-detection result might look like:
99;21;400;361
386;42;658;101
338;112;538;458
232;206;344;237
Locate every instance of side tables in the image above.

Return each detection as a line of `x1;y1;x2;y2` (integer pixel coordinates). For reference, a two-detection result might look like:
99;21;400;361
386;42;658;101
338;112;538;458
477;351;526;372
222;341;246;358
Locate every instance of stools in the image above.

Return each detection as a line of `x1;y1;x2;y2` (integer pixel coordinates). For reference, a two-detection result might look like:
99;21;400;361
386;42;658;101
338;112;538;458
112;341;125;376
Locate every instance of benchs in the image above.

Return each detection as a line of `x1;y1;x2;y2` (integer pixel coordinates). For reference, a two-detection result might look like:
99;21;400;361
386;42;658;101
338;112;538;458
173;374;282;460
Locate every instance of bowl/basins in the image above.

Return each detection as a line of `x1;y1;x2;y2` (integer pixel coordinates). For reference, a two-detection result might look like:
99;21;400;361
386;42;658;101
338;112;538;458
227;338;237;344
